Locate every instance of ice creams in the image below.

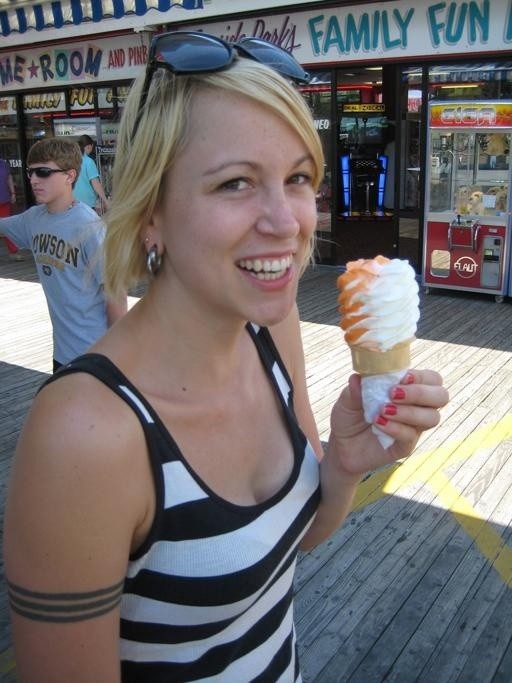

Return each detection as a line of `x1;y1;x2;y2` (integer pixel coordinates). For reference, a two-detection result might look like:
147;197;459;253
338;252;420;450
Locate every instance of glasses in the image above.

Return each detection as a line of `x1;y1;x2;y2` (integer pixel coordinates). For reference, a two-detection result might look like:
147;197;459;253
25;167;64;178
131;29;312;141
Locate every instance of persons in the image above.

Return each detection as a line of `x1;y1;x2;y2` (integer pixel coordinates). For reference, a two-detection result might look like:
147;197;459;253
0;157;20;258
0;139;128;373
72;135;110;214
3;30;448;683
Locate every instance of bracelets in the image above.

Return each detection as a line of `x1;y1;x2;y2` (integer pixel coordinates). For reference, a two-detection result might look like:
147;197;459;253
10;191;16;196
